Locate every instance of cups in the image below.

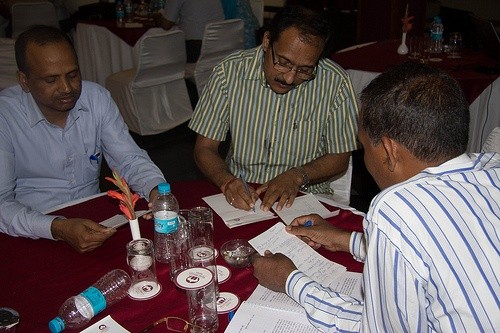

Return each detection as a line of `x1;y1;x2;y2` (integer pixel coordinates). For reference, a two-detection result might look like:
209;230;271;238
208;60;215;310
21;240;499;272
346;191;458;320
449;32;460;53
166;204;218;333
125;238;158;298
410;37;423;59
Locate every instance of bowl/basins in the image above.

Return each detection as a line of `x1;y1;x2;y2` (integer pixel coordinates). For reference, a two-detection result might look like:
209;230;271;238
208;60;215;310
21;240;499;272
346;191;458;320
220;238;257;269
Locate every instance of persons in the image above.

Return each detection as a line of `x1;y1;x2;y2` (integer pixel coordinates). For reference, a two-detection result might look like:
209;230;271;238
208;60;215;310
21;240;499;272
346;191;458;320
0;25;167;254
250;62;500;333
188;4;360;212
154;0;225;143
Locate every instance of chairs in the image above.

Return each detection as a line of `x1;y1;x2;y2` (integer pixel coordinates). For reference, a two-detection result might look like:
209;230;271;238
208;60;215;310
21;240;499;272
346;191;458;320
9;0;386;204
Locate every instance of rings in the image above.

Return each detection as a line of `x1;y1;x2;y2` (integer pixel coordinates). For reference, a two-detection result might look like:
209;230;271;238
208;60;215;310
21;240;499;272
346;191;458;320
230;198;234;204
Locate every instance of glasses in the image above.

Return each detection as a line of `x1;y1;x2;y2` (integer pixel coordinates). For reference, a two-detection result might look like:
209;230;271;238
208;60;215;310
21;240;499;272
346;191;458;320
271;35;318;81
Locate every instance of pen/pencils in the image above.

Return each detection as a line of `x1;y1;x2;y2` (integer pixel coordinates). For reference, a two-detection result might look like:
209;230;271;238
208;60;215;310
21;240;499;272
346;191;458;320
303;221;312;227
239;172;256;212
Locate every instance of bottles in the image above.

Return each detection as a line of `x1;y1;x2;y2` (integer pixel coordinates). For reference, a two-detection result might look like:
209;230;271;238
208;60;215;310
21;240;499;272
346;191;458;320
428;16;444;53
152;182;179;263
49;269;132;333
115;0;166;27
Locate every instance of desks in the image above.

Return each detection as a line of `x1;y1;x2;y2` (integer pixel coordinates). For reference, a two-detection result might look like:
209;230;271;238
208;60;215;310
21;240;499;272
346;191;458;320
0;179;366;333
327;37;500;106
78;12;158;46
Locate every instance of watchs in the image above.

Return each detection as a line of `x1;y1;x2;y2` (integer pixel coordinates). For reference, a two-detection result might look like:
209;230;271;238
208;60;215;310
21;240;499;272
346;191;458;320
291;166;310;192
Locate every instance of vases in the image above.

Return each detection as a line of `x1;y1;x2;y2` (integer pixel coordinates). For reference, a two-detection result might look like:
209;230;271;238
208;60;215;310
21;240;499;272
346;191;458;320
126;217;147;256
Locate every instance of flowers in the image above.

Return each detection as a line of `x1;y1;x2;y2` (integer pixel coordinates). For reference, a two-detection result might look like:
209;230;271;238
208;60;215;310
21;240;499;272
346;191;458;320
104;169;140;219
